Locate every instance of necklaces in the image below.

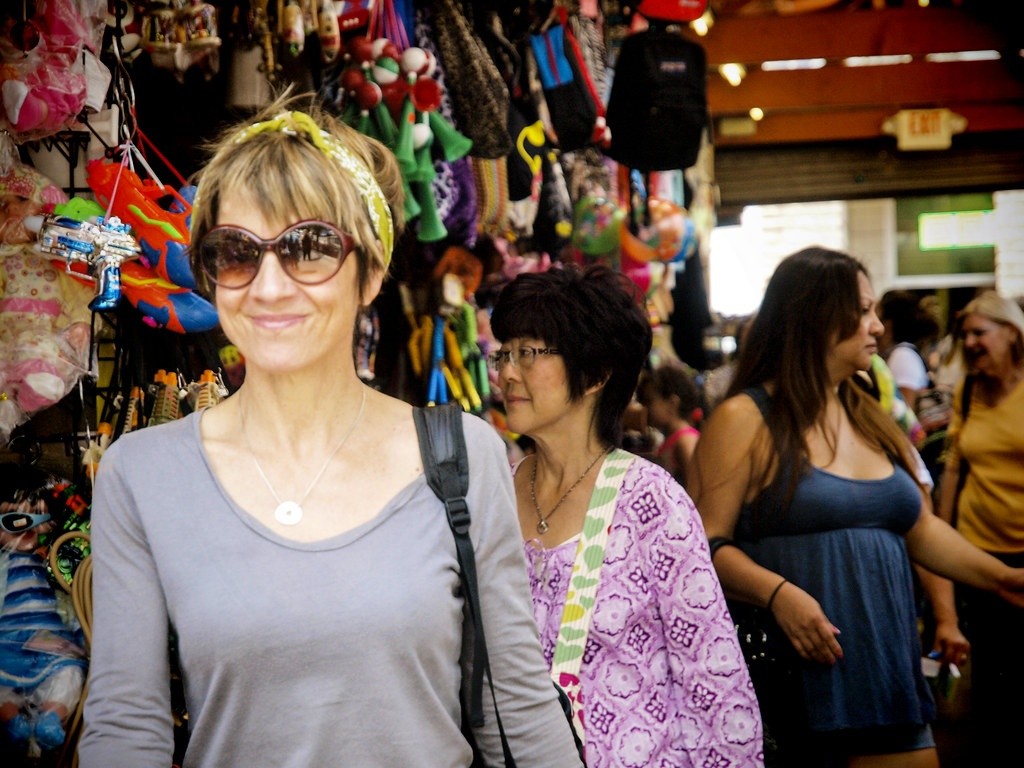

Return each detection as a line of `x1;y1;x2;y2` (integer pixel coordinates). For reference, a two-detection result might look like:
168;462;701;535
529;448;607;534
236;388;369;526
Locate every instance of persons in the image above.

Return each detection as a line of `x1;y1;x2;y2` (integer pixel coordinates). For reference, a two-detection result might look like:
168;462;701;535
638;247;1023;768
77;92;585;768
0;171;103;428
489;267;768;768
1;483;84;748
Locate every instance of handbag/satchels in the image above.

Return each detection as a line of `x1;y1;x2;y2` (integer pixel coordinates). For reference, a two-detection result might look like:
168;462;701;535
889;344;952;466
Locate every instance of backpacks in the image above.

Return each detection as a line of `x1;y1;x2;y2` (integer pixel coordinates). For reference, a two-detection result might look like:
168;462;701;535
396;0;709;226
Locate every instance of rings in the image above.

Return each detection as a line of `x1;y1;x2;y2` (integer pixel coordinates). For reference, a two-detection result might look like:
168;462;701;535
961;654;967;663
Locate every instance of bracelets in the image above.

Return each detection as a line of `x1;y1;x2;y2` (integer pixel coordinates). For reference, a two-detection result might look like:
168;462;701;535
767;579;790;612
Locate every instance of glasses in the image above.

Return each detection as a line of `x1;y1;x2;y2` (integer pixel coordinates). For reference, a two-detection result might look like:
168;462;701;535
486;346;562;371
197;219;359;290
